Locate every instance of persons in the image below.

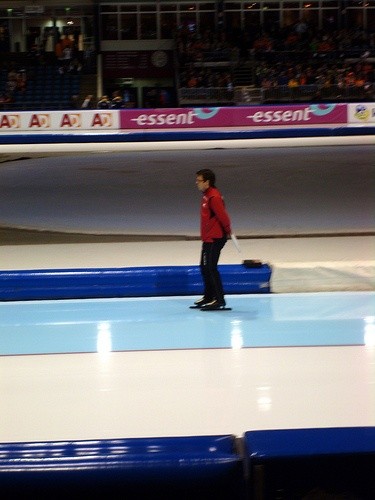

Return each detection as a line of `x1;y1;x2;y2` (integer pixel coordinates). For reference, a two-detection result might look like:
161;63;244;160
144;26;375;107
110;90;126;109
81;94;95;108
0;30;75;109
189;170;230;311
96;95;111;109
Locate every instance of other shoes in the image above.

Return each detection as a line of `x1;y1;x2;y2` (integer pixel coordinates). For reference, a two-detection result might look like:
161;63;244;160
191;296;232;311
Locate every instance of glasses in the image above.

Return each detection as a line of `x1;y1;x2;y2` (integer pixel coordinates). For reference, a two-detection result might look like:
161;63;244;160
196;178;210;183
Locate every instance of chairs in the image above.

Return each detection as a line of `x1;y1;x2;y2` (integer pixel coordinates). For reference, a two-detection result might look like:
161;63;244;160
0;49;375;110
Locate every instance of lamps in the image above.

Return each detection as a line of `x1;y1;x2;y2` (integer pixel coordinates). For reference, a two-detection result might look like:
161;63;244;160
65;17;74;25
